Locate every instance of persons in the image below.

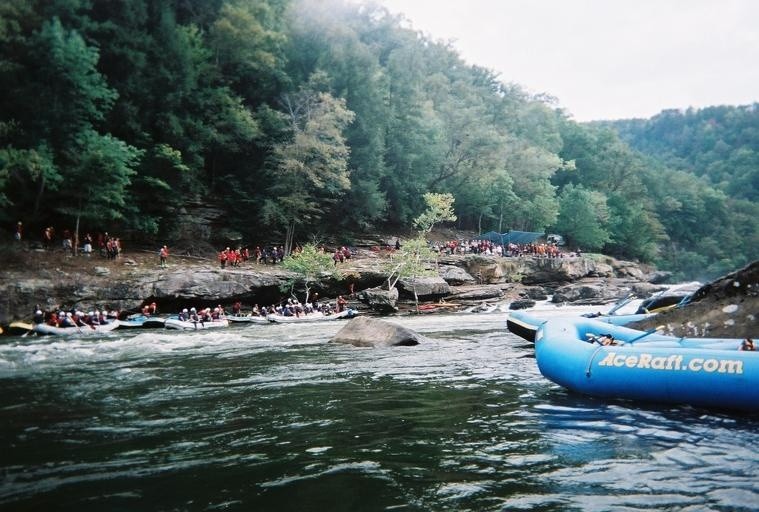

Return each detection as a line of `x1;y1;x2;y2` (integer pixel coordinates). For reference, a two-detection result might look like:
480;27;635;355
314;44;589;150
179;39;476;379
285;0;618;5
736;337;756;350
318;241;352;267
83;230;122;263
217;244;303;269
586;332;625;346
428;236;582;259
469;269;485;285
40;225;56;250
61;227;72;257
13;220;25;243
157;246;169;269
384;237;401;251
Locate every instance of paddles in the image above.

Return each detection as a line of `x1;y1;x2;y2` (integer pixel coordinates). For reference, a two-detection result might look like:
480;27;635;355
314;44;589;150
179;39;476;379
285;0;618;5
21;323;37;337
79;320;96;331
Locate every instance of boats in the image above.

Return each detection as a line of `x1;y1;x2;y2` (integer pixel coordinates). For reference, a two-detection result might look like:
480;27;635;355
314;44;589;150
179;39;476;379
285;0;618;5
532;310;759;414
505;304;661;345
